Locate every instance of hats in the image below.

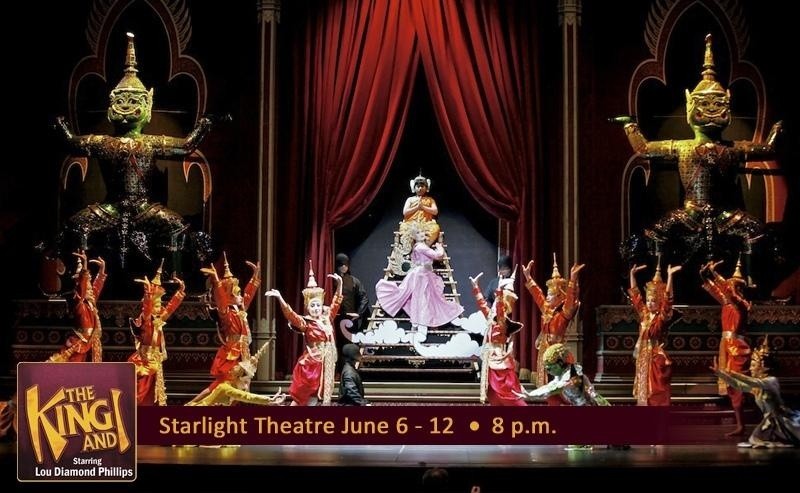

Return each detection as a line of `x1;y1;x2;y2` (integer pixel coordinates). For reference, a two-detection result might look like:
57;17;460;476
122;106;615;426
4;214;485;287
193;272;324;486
336;253;350;266
545;278;567;297
726;278;746;296
302;287;324;305
217;278;242;305
230;360;257;388
72;271;92;294
142;284;165;311
644;281;666;304
751;347;775;372
495;290;518;313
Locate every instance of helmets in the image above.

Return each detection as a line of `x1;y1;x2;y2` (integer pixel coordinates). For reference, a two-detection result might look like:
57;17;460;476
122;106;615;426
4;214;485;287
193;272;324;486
496;256;512;269
412;176;429;187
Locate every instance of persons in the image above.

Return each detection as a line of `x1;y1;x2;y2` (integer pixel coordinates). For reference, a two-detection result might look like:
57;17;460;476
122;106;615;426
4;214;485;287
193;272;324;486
376;221;466;341
126;275;187;407
700;259;757;436
41;249;107;362
200;259;263;379
183;358;289;406
468;270;529;406
265;271;345;406
603;76;786;306
334;252;369;343
522;258;586;389
708;346;800;448
510;342;613;406
338;343;371;406
46;71;212;304
626;262;682;406
484;254;513;304
401;174;441;246
